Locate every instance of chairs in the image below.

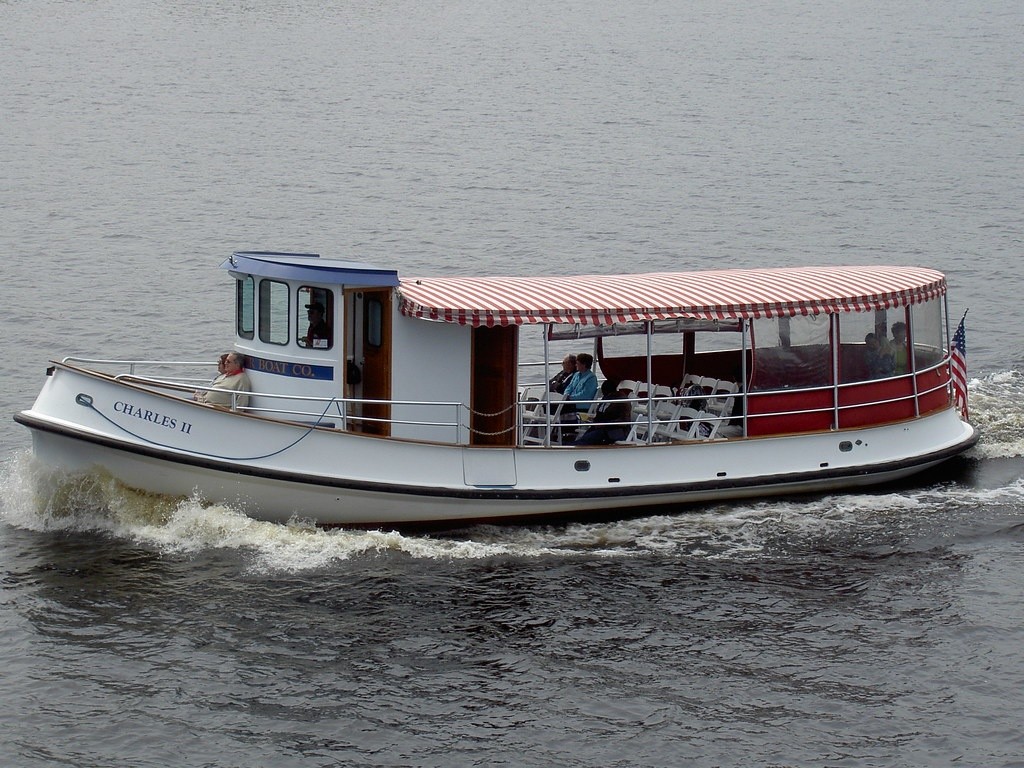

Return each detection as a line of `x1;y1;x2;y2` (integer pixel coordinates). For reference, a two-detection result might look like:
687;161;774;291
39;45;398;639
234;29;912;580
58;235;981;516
517;374;742;447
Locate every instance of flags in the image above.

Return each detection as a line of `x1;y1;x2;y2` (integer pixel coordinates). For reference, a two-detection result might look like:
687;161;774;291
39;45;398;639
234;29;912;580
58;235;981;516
944;317;969;420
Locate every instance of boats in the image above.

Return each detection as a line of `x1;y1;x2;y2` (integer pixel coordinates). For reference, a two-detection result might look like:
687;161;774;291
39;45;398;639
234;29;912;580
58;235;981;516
13;252;981;532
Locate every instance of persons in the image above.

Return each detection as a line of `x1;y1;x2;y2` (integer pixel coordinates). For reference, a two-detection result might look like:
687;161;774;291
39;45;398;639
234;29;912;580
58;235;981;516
860;322;910;379
305;302;333;348
549;352;630;447
195;352;250;412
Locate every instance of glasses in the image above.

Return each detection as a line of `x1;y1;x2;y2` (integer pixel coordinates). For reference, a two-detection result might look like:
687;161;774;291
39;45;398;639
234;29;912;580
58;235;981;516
217;360;223;364
226;359;232;364
